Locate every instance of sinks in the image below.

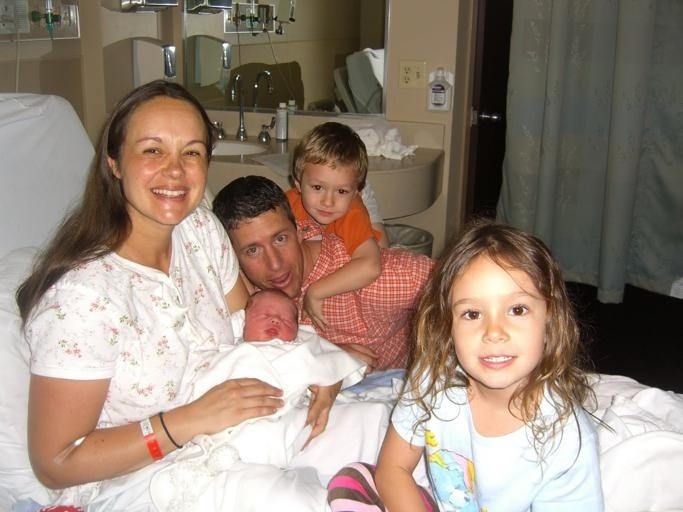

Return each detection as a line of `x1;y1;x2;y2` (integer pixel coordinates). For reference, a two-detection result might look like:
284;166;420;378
211;140;265;156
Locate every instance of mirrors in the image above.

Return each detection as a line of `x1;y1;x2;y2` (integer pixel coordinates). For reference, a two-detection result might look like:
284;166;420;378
181;0;390;119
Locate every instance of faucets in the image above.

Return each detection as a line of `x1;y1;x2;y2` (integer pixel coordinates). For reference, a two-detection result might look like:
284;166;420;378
230;74;248;141
252;70;274;106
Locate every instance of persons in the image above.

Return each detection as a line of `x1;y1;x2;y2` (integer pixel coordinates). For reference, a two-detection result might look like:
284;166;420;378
212;175;440;374
325;219;617;512
285;121;383;335
183;290;366;462
15;79;345;491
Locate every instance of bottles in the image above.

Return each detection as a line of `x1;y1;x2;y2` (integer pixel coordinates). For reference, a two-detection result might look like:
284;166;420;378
428;65;453;113
287;100;298;110
276;102;288;141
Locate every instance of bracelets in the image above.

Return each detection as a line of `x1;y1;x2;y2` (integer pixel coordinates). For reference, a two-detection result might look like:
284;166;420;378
138;417;163;462
158;411;182;449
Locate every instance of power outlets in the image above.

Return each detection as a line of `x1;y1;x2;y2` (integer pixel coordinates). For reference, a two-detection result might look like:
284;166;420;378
399;60;428;88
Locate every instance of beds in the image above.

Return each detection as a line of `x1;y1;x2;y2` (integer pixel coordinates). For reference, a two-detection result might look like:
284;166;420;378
0;92;682;512
